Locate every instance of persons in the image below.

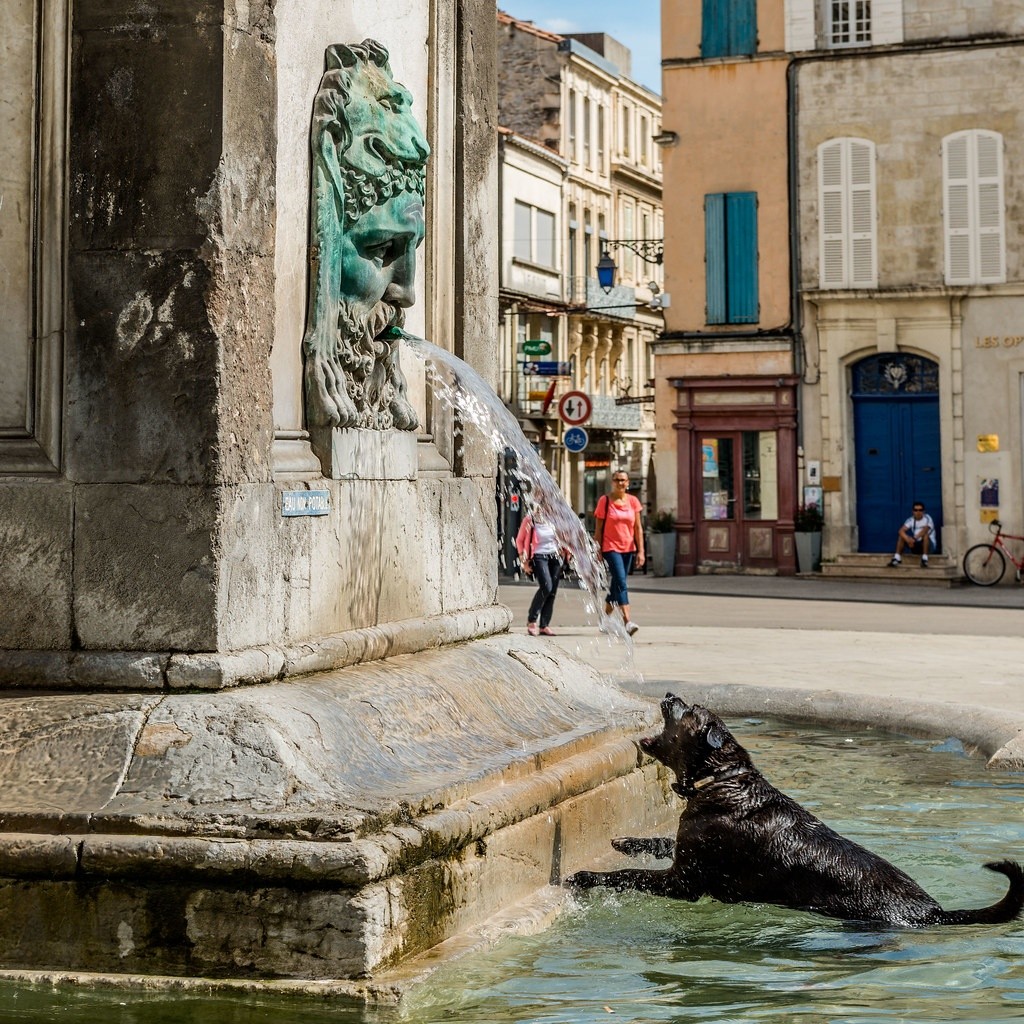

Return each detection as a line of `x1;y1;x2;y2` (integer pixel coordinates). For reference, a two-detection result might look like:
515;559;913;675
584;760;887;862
887;503;936;570
319;171;428;354
516;480;572;639
592;470;645;636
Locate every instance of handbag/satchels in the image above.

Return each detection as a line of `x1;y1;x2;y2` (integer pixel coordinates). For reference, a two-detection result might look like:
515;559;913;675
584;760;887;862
525;559;536;582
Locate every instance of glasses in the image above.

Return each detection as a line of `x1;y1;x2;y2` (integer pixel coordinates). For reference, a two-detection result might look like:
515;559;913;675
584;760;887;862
613;479;628;483
914;509;923;512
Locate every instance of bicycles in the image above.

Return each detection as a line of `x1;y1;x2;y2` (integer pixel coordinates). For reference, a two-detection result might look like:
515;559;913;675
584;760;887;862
961;518;1024;586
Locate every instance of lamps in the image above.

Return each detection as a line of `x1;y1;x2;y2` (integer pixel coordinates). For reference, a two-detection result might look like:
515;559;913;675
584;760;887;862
595;238;664;294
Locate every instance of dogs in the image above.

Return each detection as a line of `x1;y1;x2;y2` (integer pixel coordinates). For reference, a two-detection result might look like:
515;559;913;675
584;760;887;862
567;691;1024;936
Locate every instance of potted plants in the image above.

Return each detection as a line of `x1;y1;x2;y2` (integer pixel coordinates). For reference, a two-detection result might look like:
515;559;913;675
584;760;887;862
647;508;677;578
793;503;823;573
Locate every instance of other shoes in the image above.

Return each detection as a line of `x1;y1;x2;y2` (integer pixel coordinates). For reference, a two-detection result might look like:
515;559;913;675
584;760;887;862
920;559;928;568
527;622;537;636
626;623;639;637
540;628;556;636
887;558;902;567
